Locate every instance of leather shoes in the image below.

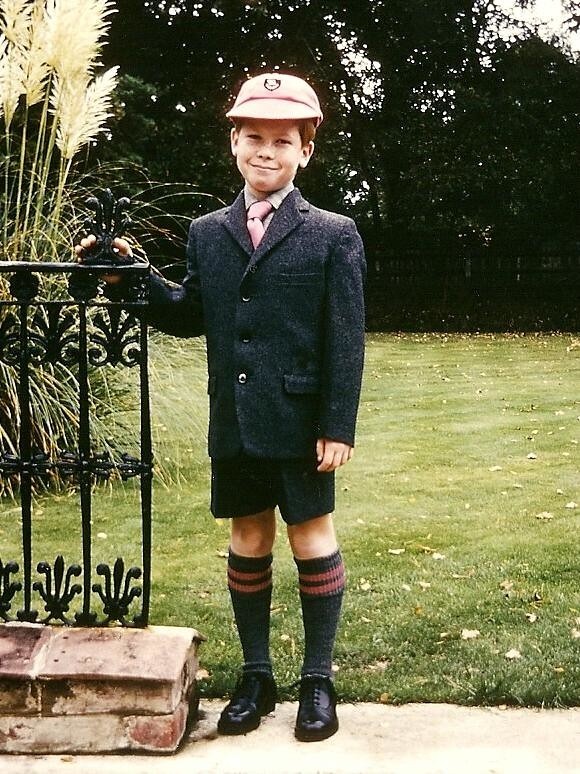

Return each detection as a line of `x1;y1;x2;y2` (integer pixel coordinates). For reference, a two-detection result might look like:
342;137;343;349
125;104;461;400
291;676;338;741
217;673;277;736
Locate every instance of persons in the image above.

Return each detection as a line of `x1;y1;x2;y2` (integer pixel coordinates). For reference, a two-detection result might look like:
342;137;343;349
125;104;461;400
75;73;366;740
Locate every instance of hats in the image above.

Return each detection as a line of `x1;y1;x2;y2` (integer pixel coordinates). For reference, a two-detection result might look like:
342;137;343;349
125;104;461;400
224;72;324;128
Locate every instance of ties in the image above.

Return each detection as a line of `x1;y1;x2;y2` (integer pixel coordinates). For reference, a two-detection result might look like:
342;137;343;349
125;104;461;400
245;199;271;250
